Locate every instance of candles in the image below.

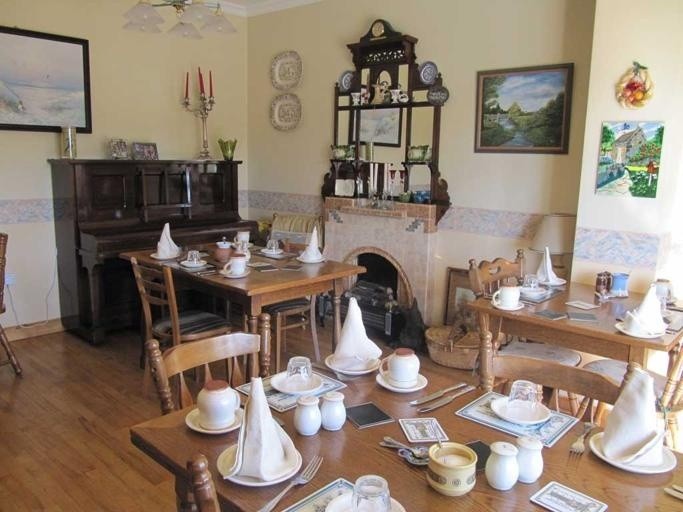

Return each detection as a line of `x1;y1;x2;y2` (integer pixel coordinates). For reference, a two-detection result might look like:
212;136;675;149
369;163;374;192
198;66;202;94
373;163;378;192
210;70;213;97
388;164;392;191
186;72;188;97
201;73;204;92
383;163;387;192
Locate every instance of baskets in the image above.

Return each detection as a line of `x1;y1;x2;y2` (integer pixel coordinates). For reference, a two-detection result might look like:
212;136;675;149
425;320;492;370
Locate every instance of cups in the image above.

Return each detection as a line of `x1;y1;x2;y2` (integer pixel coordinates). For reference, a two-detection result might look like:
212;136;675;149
524;274;539;292
188;252;200;265
656;280;673;300
379;349;420;389
196;381;241;429
352;475;392;511
506;379;539;420
223;254;246;275
285;356;314;389
268;240;278;251
236;232;246;238
492;285;521;308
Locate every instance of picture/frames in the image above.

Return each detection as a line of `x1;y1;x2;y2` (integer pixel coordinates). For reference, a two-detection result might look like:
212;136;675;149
474;63;573;153
0;24;94;133
595;121;665;200
350;106;403;147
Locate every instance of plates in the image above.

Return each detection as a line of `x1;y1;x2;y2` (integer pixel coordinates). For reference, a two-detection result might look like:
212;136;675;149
539;278;567;284
270;51;301;90
520;287;544;294
296;255;327;261
339;70;354;91
418;61;437;84
270;371;323;393
270;94;302;131
150;253;180;258
217;443;300;486
325;353;380;373
663;296;678;304
180;260;206;266
491;299;524;309
615;322;663;338
375;371;427;391
491;397;552;423
220;269;250;276
589;432;677;473
231;242;253;247
427;87;448;105
186;407;245;433
325;492;406;512
262;248;283;254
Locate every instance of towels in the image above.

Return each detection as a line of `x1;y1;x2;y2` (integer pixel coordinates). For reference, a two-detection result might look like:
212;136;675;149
331;297;382;371
223;377;298;482
599;366;665;465
300;226;322;261
623;284;666;335
157;223;179;258
537;246;559;282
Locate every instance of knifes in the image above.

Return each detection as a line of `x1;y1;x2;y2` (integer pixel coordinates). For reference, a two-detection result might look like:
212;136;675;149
408;382;467;405
417;385;476;412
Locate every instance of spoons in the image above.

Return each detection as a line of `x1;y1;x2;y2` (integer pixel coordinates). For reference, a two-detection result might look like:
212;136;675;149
378;436;429;458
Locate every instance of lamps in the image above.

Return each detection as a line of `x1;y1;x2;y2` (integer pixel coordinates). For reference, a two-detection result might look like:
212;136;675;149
528;215;577;278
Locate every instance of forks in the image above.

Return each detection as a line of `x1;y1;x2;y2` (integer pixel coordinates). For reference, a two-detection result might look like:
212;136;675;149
567;421;596;454
257;456;324;512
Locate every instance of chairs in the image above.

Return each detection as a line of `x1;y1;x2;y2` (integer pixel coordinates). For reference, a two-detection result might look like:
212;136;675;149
468;249;578;416
0;232;23;374
576;335;683;445
267;236;328;373
129;256;232;382
144;312;272;414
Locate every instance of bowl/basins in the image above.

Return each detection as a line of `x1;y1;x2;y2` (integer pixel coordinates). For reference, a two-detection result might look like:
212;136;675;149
217;242;231;248
426;443;477;496
